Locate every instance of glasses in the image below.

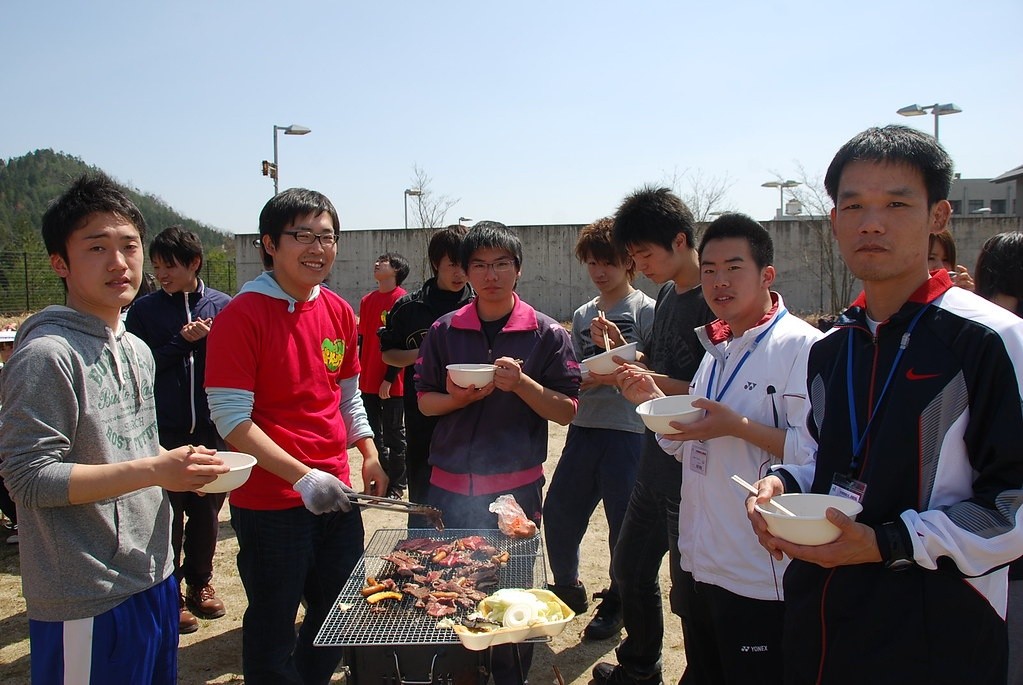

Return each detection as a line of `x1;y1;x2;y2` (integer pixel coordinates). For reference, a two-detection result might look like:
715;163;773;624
470;260;516;274
281;229;339;246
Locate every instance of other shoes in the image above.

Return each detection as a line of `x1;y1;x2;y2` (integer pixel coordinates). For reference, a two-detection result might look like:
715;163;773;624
359;485;404;505
7;523;19;543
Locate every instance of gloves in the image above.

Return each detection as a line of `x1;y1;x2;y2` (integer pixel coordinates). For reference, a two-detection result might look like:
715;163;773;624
293;468;358;515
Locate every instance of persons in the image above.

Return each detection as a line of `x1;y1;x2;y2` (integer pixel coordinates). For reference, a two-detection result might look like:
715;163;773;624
0;174;229;685
126;225;230;634
361;187;1023;685
205;188;390;685
742;125;1023;685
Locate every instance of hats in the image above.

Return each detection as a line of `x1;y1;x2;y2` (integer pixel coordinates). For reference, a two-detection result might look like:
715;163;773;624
0;331;17;342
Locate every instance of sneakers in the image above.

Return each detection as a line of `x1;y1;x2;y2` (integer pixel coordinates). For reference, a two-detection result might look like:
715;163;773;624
549;581;588;611
585;590;625;639
593;662;664;685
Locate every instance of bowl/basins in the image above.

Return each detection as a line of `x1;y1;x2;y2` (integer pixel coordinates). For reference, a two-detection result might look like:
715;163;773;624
755;493;863;546
195;451;258;494
581;342;639;376
636;394;709;434
446;363;497;389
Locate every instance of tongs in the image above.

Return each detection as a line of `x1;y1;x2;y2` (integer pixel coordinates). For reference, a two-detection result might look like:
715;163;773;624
344;493;443;518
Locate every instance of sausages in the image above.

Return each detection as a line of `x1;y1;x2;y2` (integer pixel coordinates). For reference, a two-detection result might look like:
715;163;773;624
432;551;447;563
500;552;510;562
362;584;385;596
432;592;458;597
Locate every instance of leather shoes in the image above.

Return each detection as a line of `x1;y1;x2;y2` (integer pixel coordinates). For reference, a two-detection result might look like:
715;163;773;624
187;583;226;617
179;593;198;634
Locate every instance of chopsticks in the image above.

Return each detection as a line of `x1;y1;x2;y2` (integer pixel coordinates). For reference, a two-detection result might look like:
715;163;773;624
188;444;199;455
482;359;524;369
629;369;669;379
731;475;797;517
599;310;611;352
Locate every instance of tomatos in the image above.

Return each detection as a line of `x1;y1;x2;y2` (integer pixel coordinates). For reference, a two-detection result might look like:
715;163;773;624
455;576;466;587
